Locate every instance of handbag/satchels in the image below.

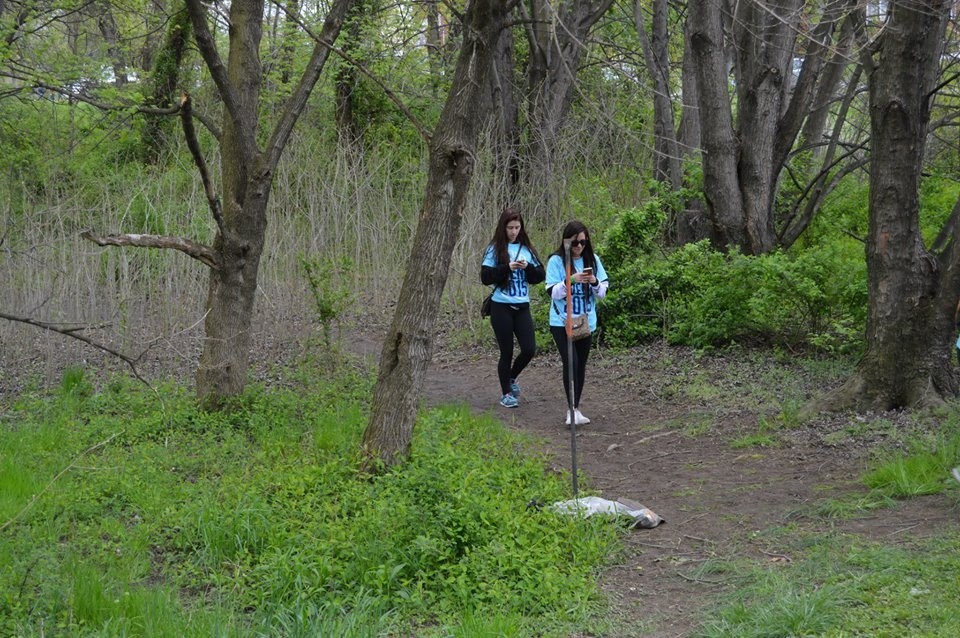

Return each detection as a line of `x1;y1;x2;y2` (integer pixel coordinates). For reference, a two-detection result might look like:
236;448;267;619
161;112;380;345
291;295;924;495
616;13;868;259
481;294;493;319
564;314;591;342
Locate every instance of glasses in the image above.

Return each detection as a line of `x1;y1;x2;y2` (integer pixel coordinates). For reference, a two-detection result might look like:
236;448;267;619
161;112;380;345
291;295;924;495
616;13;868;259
571;239;589;247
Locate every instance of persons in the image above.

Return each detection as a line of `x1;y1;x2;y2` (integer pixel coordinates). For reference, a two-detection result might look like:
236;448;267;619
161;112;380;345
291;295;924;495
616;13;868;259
480;209;546;408
545;221;608;425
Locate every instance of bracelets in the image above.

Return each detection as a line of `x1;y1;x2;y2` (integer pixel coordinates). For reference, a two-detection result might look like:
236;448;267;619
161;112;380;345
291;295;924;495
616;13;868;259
570;275;577;285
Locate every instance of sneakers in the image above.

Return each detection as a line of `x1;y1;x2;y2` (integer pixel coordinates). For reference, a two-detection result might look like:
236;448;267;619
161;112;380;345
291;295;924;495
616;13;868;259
565;410;578;425
500;392;519;408
510;378;521;397
577;410;591;424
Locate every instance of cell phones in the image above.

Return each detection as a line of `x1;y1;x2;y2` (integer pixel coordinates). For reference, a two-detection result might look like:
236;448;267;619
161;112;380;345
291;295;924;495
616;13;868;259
517;260;524;263
583;268;593;276
